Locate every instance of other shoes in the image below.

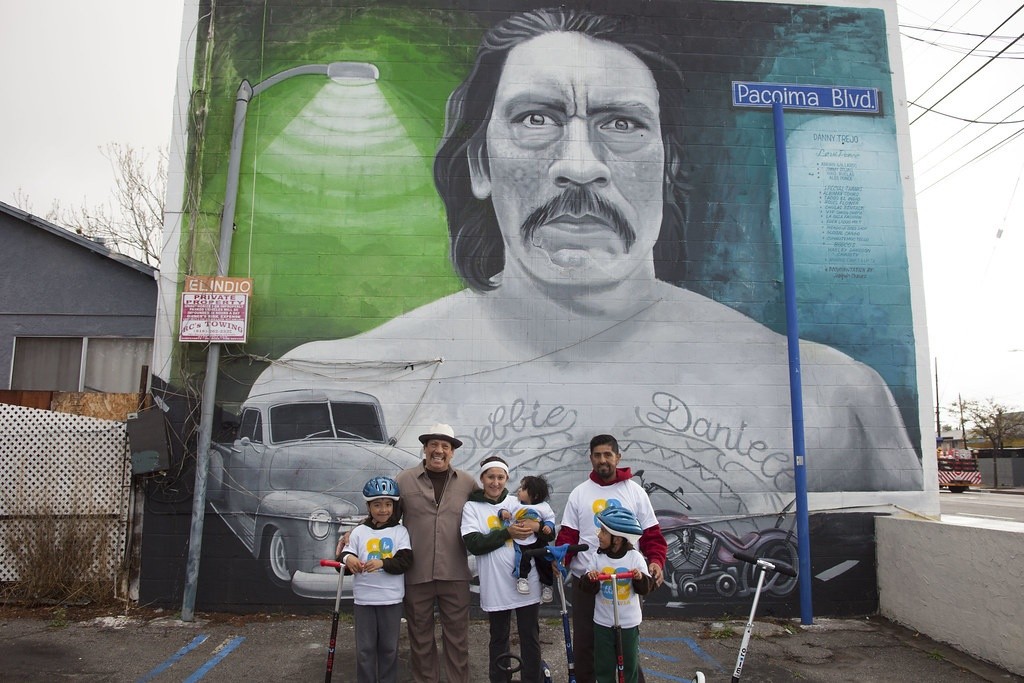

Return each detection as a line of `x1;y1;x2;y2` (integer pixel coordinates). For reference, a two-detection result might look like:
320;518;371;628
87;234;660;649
517;578;529;594
540;585;554;602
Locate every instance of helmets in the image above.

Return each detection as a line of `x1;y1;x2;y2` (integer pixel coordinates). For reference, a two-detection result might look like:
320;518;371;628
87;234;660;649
596;506;643;545
362;476;401;501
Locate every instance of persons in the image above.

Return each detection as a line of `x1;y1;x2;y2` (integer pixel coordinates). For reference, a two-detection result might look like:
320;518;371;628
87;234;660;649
335;423;668;683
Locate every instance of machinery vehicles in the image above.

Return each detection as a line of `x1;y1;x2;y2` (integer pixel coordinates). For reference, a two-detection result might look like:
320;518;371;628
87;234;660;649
937;435;982;493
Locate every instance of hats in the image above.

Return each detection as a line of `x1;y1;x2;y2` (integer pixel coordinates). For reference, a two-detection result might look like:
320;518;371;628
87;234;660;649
418;423;463;448
